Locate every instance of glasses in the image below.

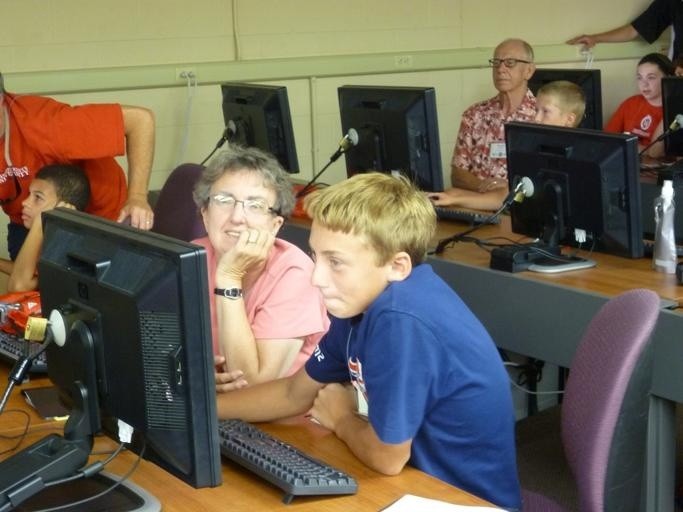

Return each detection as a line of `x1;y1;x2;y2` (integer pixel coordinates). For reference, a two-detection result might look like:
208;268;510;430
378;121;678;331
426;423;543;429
204;193;286;215
487;57;531;68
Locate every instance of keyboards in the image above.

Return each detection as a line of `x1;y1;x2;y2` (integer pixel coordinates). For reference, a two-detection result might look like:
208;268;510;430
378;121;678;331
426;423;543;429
219;420;357;505
431;206;499;227
0;333;47;372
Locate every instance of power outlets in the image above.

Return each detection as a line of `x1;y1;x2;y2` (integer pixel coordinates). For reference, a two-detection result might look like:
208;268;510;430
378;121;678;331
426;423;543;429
175;66;197;82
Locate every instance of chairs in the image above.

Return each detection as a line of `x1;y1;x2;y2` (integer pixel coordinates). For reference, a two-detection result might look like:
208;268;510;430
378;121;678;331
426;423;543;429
520;286;663;510
146;161;213;246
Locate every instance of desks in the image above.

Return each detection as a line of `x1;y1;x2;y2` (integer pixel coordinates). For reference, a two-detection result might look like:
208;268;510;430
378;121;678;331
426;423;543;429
635;146;682;269
1;353;504;512
280;180;681;511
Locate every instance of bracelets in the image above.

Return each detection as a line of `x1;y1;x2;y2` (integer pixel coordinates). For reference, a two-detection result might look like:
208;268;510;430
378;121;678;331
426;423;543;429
213;288;244;299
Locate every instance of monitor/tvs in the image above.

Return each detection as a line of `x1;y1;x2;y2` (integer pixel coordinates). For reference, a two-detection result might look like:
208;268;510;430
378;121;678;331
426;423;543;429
221;82;300;174
527;67;603;130
0;205;221;512
504;122;642;272
661;76;683;157
338;85;443;194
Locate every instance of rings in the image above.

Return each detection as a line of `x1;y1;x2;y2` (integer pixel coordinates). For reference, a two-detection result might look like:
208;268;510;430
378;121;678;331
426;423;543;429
249;237;258;244
145;221;151;223
492;180;499;185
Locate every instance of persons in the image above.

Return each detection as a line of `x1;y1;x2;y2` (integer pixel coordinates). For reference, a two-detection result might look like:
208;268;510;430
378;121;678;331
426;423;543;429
566;0;683;161
0;72;155;261
0;166;89;295
188;140;331;436
450;38;536;192
424;79;586;211
216;170;523;511
